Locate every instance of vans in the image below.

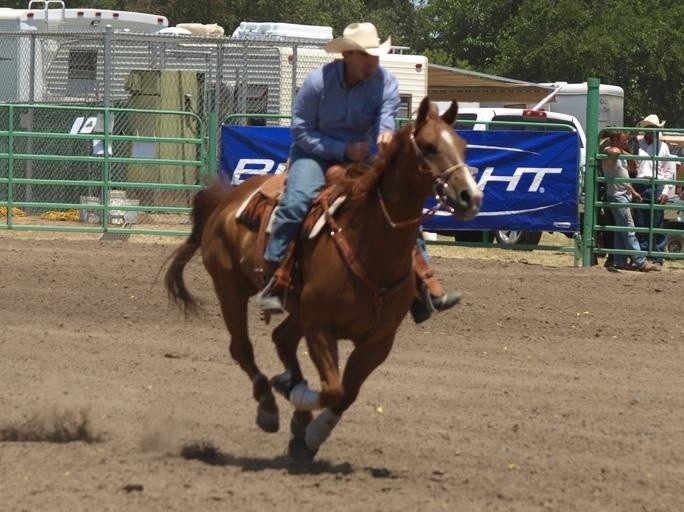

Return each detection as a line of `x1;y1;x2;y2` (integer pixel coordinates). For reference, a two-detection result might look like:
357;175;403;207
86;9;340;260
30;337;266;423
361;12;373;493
421;107;590;252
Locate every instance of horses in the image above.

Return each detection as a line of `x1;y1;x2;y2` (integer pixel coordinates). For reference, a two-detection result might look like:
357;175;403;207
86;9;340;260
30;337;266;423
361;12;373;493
597;130;640;266
142;96;484;464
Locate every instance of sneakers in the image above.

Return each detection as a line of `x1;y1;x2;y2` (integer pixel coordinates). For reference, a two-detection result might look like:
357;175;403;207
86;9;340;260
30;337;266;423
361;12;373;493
618;259;661;272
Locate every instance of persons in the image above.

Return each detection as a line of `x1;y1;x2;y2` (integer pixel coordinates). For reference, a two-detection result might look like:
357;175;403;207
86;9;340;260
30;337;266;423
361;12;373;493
257;22;466;316
602;131;661;272
630;114;674;266
247;116;266;126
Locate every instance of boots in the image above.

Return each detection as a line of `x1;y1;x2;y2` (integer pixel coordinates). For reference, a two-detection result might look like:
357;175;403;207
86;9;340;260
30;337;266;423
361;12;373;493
410;276;460;323
256;263;284;313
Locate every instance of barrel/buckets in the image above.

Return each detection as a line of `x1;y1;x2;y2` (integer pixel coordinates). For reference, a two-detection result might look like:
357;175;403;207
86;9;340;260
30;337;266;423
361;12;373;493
80;190;140;224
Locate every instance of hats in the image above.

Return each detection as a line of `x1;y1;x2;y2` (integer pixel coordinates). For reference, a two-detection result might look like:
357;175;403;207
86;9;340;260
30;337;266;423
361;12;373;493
639;114;666;128
324;22;391;57
